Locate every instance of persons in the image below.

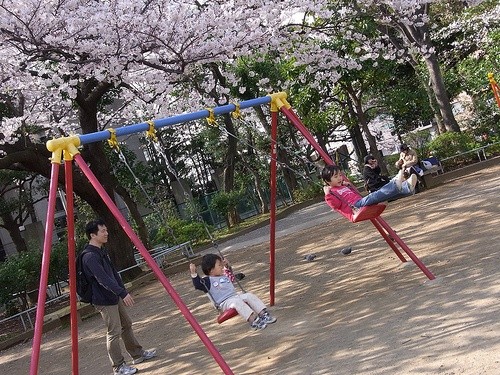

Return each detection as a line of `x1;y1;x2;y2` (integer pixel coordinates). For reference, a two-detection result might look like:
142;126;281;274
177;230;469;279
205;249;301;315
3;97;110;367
189;253;278;334
81;219;157;375
362;156;390;193
321;165;416;223
395;144;418;180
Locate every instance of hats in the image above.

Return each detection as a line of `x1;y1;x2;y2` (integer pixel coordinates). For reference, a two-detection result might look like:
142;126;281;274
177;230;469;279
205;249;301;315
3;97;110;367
399;144;409;152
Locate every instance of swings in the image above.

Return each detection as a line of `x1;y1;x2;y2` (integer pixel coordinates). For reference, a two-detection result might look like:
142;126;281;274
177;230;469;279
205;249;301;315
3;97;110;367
108;131;251;324
207;112;386;222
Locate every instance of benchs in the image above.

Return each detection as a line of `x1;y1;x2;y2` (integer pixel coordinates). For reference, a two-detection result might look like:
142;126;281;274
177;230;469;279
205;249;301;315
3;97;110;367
416;166;442;187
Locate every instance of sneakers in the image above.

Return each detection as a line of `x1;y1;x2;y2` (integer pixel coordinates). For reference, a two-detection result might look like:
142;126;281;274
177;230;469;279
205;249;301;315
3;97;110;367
133;348;157;364
114;363;138;375
394;170;403;190
260;311;277;324
251;316;267;332
407;174;417;192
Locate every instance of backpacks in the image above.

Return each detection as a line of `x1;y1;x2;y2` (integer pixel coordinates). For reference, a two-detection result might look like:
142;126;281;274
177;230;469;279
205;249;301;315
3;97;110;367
76;249;104;300
